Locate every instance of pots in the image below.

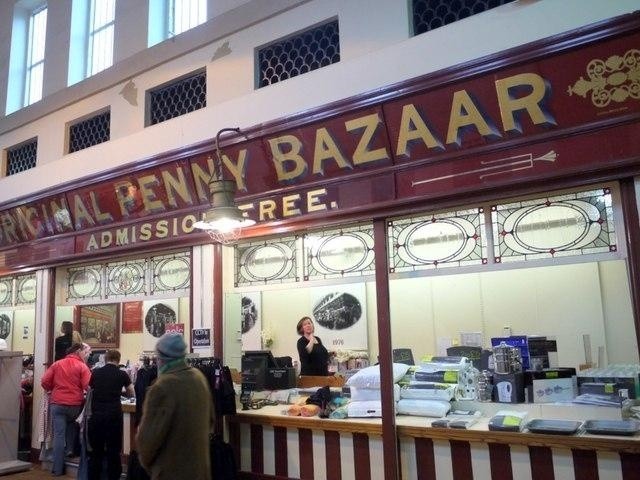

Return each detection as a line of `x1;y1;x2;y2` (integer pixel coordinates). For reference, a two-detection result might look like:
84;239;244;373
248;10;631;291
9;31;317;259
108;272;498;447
490;341;515;375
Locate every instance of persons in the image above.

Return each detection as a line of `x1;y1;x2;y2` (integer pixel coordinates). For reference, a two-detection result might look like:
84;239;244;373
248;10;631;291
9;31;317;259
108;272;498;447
296;317;338;376
41;321;135;480
134;333;217;480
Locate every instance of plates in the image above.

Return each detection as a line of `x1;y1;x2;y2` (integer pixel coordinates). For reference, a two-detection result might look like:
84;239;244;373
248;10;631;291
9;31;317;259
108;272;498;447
523;418;639;435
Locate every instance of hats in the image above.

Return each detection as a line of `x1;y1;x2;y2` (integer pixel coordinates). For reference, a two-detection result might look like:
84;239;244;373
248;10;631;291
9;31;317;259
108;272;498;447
155;332;186;359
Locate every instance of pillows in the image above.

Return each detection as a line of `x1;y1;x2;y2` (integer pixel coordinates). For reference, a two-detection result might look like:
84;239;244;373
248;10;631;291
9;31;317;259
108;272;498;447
345;363;410;390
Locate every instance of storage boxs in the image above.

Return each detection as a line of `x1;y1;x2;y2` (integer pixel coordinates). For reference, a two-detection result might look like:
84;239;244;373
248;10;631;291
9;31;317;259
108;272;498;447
532;367;579;403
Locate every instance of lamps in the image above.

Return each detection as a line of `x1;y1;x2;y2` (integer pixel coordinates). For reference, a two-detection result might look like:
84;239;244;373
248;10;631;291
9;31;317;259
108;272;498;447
192;127;256;245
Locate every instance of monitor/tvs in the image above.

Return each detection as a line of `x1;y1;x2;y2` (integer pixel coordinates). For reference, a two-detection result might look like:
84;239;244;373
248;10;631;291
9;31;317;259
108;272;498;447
245;350;279;369
